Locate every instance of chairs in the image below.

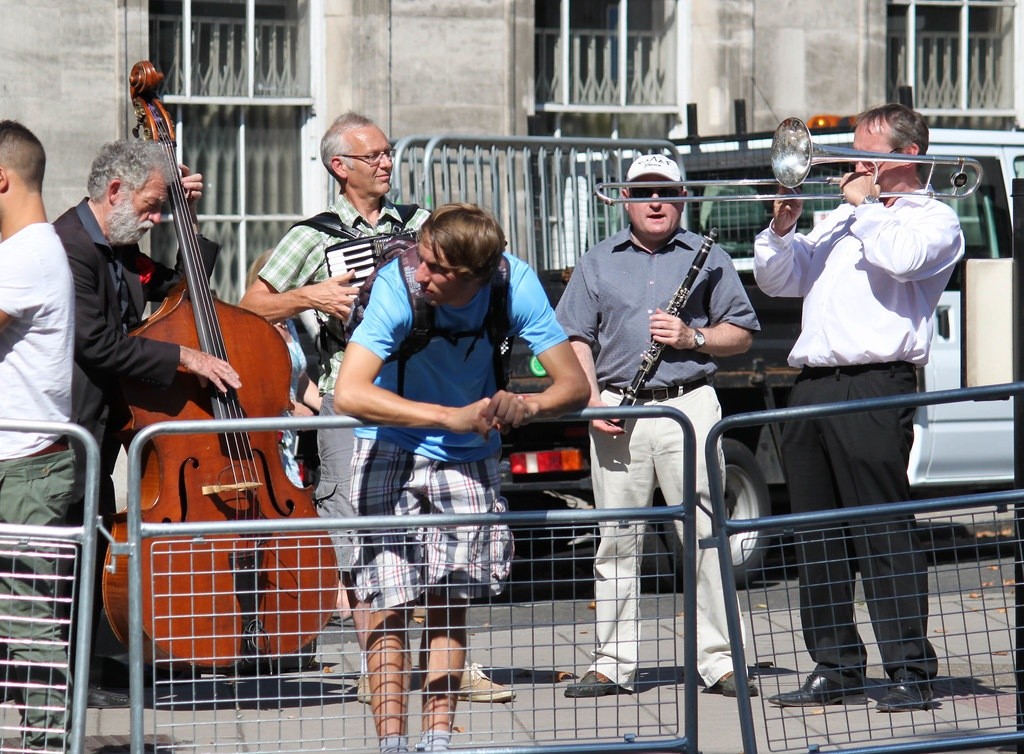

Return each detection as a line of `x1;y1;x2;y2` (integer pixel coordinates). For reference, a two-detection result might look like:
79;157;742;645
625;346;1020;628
706;184;766;255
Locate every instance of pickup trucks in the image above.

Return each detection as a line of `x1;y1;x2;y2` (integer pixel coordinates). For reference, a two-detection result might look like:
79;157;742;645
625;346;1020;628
295;86;1024;588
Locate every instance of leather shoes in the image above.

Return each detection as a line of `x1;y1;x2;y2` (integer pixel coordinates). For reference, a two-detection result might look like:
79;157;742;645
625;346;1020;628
875;675;935;711
768;675;868;707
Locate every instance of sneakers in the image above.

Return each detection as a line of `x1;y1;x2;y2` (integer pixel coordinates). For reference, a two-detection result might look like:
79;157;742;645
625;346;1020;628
457;663;516;704
560;672;630;698
358;676;372;705
698;672;758;698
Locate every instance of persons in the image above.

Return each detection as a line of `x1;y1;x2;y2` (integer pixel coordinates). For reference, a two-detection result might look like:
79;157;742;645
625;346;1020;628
51;139;241;707
754;106;966;711
244;248;362;621
332;202;591;754
0;119;76;754
557;154;760;698
238;113;517;702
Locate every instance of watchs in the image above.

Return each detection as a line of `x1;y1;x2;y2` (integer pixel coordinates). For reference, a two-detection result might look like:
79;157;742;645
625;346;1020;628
861;195;880;204
691;328;705;350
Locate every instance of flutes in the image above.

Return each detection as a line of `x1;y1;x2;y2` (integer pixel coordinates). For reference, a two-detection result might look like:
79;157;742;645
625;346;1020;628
604;226;721;433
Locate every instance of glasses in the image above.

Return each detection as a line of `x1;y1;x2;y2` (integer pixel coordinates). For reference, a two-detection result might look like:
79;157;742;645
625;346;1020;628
341;152;398;164
628;187;680;198
849;146;901;171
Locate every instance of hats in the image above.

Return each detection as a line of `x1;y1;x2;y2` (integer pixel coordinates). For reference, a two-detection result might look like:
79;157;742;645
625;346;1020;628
622;153;684;184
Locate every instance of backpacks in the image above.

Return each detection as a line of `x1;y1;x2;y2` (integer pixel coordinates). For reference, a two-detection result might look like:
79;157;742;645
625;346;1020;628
348;237;516;387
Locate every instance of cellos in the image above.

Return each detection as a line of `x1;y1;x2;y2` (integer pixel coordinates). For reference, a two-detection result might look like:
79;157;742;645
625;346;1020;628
101;61;341;671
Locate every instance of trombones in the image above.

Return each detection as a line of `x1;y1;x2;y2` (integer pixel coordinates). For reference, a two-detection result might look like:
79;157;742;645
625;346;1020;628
593;116;985;205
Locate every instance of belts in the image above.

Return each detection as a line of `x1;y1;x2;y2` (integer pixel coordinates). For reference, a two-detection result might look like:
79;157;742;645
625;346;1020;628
25;434;70;458
607;375;709;402
